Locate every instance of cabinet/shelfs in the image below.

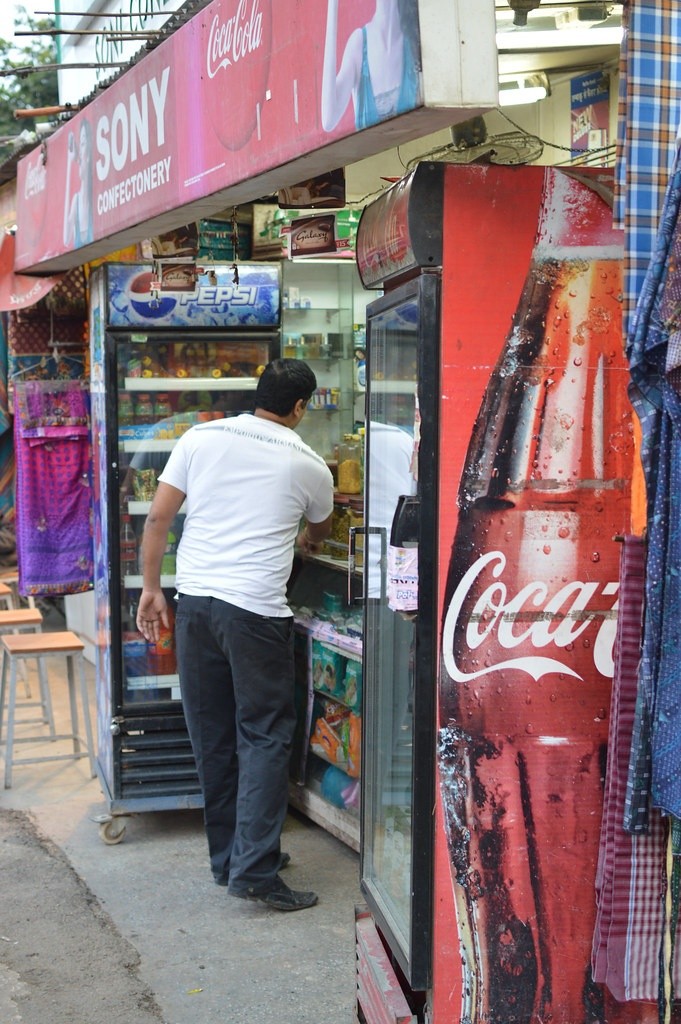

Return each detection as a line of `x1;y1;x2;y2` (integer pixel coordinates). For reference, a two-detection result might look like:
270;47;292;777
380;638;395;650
286;492;365;854
278;257;385;465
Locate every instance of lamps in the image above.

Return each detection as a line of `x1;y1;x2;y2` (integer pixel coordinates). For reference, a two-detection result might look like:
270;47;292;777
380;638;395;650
498;78;551;107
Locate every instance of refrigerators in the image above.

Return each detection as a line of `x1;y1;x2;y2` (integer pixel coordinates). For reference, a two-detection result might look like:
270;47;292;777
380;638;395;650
88;255;284;811
349;162;659;1024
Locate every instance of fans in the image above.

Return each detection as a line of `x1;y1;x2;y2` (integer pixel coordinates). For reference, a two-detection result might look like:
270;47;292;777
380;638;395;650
406;116;545;167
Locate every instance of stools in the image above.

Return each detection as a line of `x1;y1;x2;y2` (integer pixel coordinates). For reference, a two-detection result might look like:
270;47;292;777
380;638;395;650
1;572;97;790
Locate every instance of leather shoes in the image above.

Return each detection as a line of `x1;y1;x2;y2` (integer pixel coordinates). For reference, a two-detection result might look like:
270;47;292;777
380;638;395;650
226;881;318;911
213;852;291;886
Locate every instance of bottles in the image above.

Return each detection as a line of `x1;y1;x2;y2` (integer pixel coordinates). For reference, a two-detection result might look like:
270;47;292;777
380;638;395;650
122;591;179;677
121;515;183;577
118;395;133;426
438;167;660;1024
155;394;173;425
128;344;265;378
339;428;364;494
136;394;154;426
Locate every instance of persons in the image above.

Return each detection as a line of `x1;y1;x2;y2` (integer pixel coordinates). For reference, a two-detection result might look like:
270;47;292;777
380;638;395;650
321;0;422;133
63;117;95;250
136;358;334;913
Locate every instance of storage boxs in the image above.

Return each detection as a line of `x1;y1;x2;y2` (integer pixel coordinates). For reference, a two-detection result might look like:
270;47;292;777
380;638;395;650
301;334;325;357
353;324;366;349
284;287;312;308
328;333;343;358
